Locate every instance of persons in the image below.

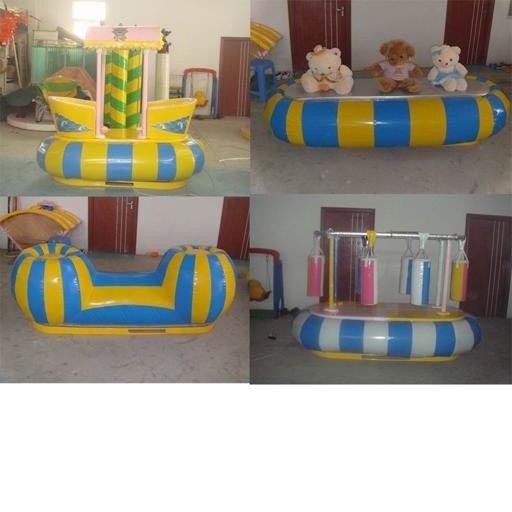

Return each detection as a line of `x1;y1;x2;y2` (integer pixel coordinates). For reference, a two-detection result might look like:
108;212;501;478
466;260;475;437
300;45;354;96
